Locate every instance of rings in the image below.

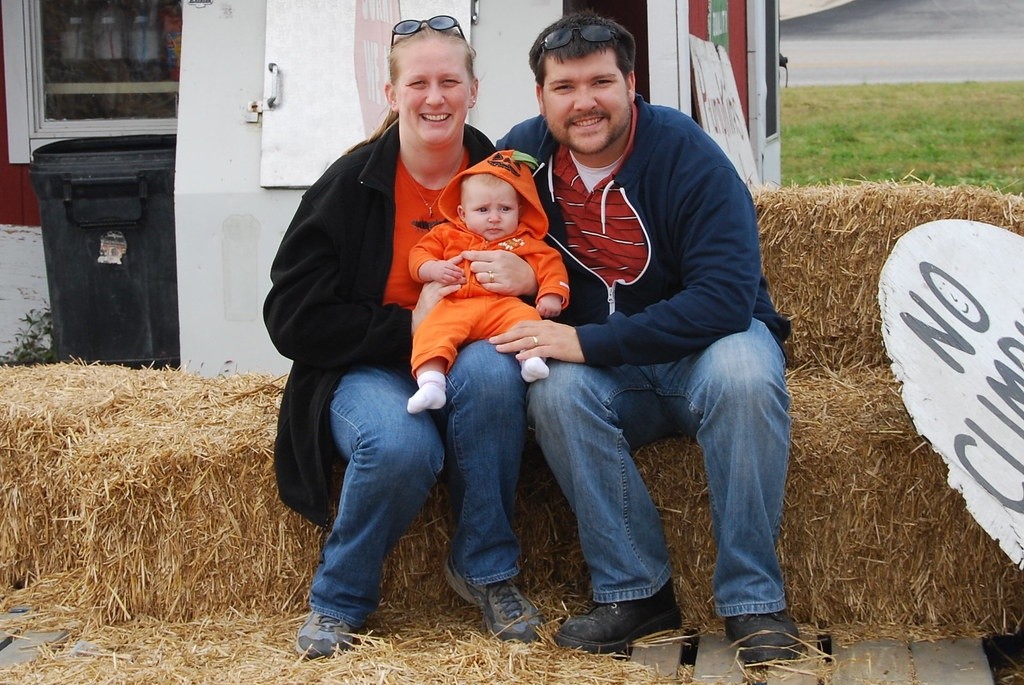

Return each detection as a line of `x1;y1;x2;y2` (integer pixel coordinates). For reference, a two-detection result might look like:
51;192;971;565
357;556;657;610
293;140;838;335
488;272;495;281
533;336;537;346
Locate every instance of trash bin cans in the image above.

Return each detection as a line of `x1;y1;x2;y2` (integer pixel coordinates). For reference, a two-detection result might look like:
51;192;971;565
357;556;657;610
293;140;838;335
28;132;182;371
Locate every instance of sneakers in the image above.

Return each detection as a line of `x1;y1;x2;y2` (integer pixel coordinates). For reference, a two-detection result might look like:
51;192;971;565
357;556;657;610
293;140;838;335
295;612;354;660
554;577;682;654
443;550;546;645
725;609;800;662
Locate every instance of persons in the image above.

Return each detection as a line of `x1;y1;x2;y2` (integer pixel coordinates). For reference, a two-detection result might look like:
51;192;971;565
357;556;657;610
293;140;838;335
488;16;802;665
408;150;569;414
265;16;543;665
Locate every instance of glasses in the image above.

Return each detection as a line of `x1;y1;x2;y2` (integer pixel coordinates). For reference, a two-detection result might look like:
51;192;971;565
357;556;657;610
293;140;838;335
391;15;469;53
535;25;630;74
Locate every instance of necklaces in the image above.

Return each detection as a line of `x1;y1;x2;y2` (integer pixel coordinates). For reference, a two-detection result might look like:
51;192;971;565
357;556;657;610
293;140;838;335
405;159;459;216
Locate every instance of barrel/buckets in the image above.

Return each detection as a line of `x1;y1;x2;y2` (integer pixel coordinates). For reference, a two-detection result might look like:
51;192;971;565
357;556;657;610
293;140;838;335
26;135;180;372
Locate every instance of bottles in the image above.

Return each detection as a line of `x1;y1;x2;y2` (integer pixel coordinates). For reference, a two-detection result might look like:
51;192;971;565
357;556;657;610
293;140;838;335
128;15;161;71
162;0;184;82
93;16;123;60
60;15;85;66
